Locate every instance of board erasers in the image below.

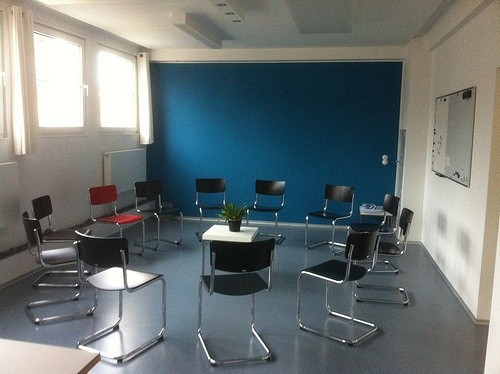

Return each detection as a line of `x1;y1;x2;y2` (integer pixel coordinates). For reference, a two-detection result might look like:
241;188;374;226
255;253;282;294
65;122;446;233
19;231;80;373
463;90;471;99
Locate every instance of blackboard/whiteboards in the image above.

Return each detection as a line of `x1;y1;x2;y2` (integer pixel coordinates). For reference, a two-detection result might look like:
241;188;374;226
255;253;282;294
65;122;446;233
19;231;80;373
432;85;476;188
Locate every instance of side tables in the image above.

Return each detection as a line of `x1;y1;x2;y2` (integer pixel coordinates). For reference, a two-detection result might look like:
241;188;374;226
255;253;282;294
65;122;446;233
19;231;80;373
202;224;259;276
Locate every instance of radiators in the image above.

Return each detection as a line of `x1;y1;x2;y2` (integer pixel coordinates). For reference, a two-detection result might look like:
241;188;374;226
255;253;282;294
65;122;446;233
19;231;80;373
103;148;146;193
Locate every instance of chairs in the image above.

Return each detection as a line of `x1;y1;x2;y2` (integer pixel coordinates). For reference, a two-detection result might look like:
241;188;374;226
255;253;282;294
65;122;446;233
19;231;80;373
347;194;400;273
305;185;355;255
195;177;226;242
296;222;382;347
134;180;184;250
76;230;166;365
89;185;145;255
31;195;91;288
248;180;286;244
22;211;98;325
353;208;415;304
196;237;276;366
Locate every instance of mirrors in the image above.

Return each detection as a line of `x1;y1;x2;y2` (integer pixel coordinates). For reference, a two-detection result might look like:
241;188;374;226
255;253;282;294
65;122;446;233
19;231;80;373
431;84;478;188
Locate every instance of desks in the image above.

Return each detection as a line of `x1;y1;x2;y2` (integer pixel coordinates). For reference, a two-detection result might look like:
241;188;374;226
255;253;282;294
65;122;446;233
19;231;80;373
0;338;101;374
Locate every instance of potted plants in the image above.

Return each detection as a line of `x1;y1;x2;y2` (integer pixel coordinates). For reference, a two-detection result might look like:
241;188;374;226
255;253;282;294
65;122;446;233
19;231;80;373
219;202;248;231
